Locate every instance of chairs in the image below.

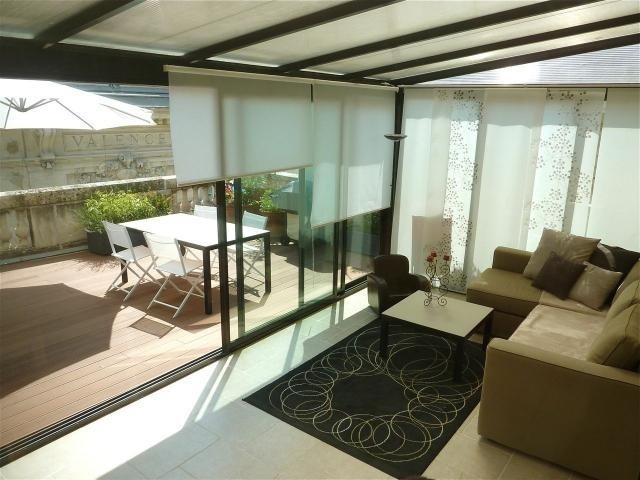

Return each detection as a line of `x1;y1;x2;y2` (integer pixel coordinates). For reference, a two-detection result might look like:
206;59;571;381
366;252;432;316
101;204;271;319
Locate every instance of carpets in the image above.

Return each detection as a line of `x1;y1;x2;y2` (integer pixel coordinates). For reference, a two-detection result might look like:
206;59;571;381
242;315;487;480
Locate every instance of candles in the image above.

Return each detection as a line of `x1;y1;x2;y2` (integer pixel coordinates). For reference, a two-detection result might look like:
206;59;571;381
425;249;451;267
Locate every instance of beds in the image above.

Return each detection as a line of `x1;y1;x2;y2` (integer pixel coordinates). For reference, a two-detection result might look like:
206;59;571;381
466;246;640;480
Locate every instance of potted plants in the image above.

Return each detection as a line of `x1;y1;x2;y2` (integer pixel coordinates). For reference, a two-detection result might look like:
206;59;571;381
70;188;175;256
220;171;296;245
344;209;382;257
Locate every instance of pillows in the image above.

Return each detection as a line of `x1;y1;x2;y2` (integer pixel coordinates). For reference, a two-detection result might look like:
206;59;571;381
521;227;640;313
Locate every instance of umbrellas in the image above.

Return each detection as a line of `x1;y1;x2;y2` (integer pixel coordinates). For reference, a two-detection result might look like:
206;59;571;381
0;79;156;131
